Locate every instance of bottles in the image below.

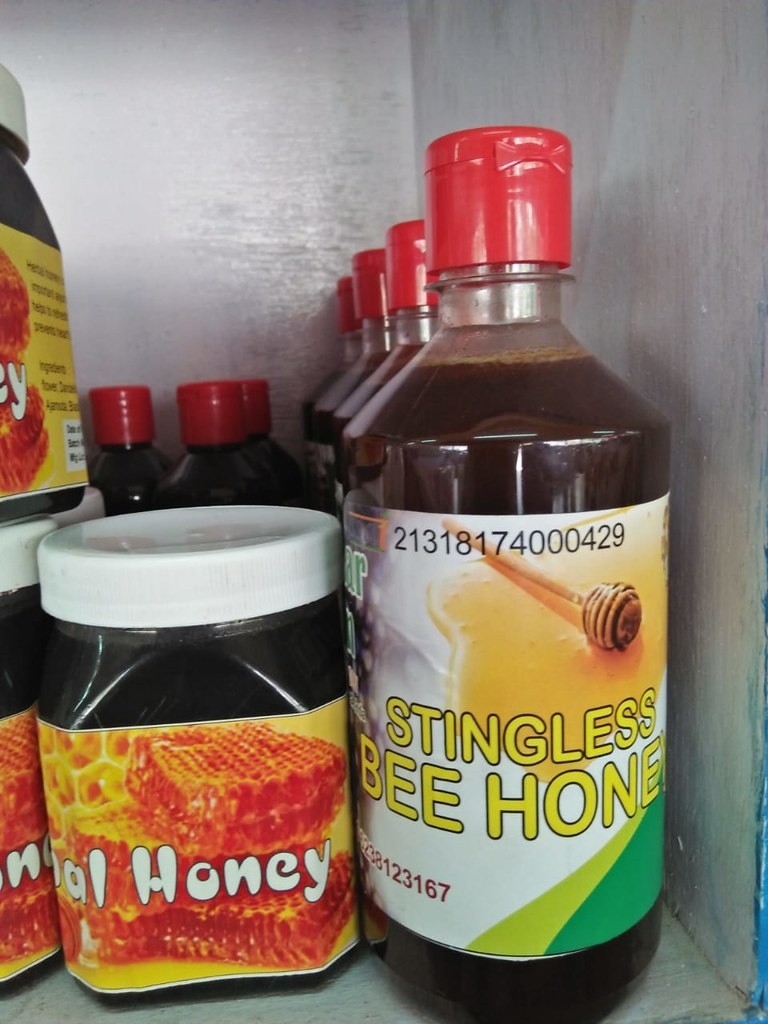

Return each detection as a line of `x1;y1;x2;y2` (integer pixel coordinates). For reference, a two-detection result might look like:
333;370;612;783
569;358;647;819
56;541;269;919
297;121;676;1024
84;373;307;521
